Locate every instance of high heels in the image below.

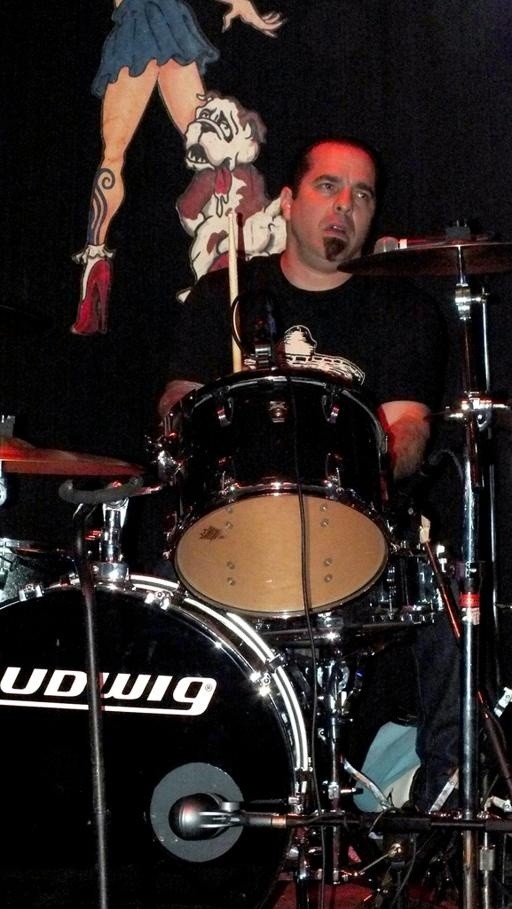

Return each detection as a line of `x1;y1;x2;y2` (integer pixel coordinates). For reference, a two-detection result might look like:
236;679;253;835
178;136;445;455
71;259;112;336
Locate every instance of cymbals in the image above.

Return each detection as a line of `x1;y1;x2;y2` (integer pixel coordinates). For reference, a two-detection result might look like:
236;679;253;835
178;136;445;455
337;242;512;276
0;437;144;475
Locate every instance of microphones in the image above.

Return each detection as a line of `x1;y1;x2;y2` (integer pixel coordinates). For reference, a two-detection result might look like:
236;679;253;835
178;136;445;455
373;235;489;256
390;455;437;509
261;305;279;365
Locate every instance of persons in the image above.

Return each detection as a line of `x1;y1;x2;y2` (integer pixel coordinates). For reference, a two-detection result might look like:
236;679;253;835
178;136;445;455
70;0;283;336
157;134;477;812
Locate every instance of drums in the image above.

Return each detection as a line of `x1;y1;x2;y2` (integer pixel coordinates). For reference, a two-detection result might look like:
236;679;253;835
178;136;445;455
0;574;309;909
143;366;388;620
254;548;438;645
